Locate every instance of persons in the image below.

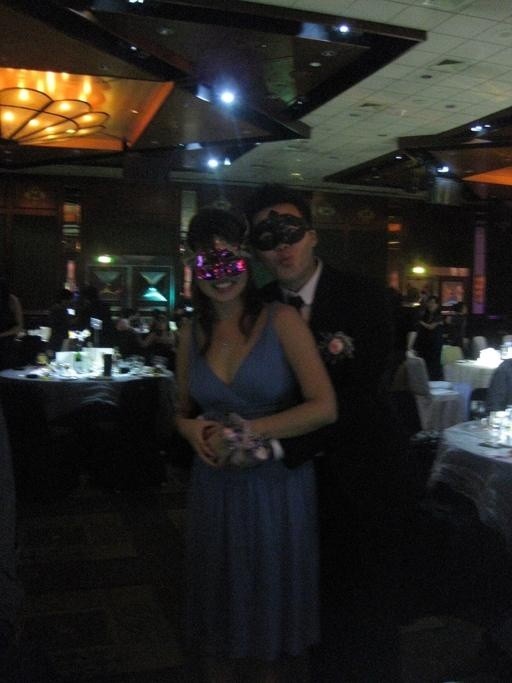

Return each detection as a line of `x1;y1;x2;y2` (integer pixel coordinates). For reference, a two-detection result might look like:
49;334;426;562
403;286;510;375
224;181;409;682
2;278;191;370
172;205;344;681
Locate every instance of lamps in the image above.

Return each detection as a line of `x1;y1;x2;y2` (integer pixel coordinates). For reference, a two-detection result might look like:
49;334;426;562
0;0;178;157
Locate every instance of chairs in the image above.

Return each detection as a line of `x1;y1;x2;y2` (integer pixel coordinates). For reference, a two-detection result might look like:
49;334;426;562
388;331;512;431
